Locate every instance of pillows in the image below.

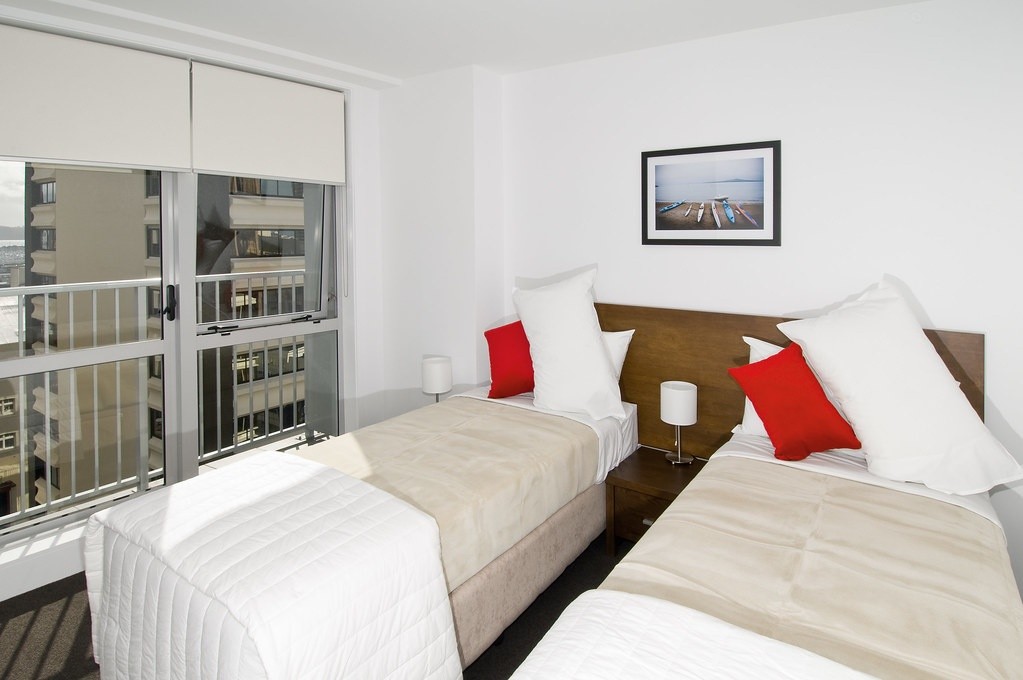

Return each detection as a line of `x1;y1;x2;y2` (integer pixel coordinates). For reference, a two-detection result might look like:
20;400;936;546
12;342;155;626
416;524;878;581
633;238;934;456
730;335;867;459
602;329;636;382
725;345;861;461
483;318;534;399
514;264;627;421
775;269;1023;494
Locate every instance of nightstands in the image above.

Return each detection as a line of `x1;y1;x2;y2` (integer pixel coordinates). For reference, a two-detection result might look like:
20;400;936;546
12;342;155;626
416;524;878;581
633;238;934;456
603;448;711;557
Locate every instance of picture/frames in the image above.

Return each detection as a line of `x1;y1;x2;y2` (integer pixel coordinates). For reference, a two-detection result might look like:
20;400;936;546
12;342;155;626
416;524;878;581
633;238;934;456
641;139;782;244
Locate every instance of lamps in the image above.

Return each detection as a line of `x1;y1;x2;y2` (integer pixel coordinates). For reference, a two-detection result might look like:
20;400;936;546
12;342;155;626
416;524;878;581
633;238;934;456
659;380;698;465
420;358;452;402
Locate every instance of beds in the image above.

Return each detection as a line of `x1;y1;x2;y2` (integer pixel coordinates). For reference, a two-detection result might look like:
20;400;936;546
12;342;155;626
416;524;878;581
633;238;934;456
83;303;1022;680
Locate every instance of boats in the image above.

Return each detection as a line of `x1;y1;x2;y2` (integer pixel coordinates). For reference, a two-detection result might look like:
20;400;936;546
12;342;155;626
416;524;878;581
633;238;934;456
736;204;760;227
697;202;704;223
723;200;734;223
712;202;721;228
660;200;685;213
714;195;729;201
685;203;692;217
